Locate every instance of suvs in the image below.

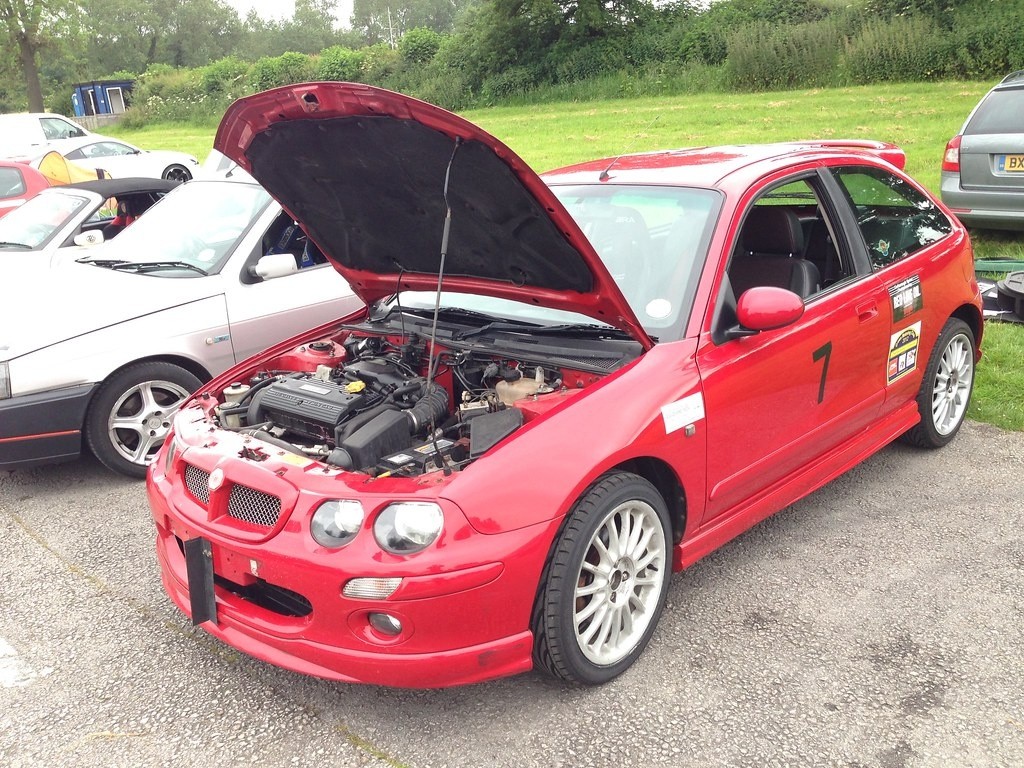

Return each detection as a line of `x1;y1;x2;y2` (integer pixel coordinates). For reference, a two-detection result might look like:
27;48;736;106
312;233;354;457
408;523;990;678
940;69;1024;232
0;167;367;479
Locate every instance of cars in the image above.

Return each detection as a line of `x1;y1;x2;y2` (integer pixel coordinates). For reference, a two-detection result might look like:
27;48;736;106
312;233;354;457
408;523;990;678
142;78;985;690
0;113;247;253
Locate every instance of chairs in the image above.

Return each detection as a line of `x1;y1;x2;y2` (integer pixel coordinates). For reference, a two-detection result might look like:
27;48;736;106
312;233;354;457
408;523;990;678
726;202;909;330
